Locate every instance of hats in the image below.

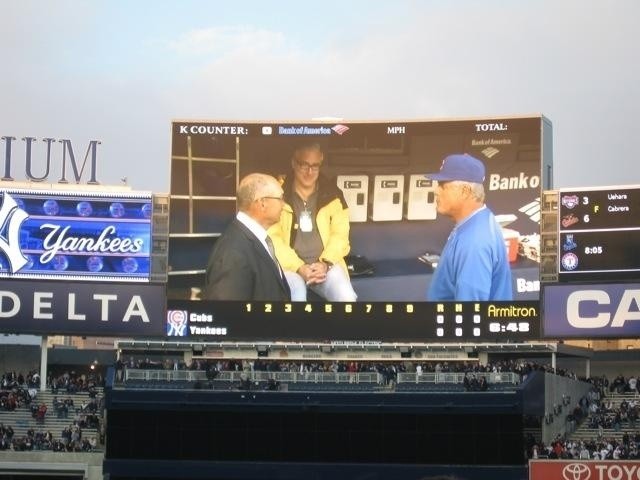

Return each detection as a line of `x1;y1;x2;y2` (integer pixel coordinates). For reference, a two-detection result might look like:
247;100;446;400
425;153;486;184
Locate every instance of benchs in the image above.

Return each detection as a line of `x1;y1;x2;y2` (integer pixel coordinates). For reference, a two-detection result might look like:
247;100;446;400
0;384;104;451
567;392;640;441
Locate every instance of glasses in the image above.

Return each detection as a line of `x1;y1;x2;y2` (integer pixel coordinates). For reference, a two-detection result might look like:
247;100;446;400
297;161;320;170
255;194;285;201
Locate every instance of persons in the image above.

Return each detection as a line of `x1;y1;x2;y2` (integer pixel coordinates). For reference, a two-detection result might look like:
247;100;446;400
529;355;640;461
203;172;292;303
117;356;530;394
0;355;108;454
423;152;513;302
265;140;359;302
